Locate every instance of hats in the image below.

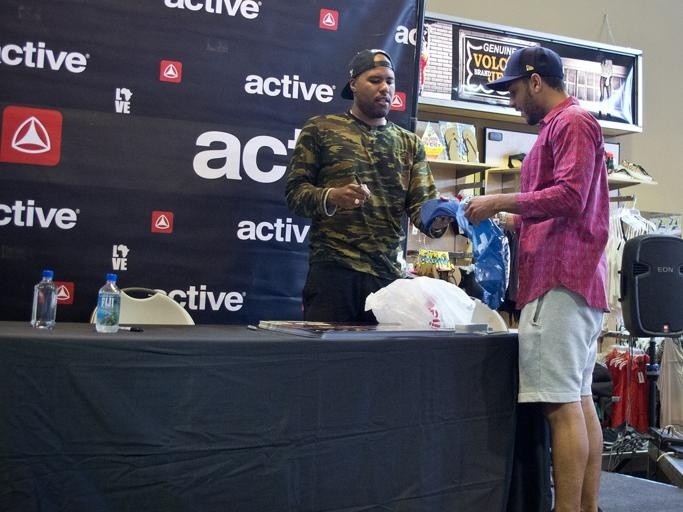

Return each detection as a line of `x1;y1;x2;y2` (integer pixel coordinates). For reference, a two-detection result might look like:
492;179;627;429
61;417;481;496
339;48;396;100
485;45;564;92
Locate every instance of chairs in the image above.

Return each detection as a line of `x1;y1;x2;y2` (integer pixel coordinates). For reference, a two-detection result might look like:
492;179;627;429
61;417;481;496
88;287;195;326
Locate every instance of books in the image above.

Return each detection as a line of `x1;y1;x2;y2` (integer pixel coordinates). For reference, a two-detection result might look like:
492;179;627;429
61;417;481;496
258;319;458;339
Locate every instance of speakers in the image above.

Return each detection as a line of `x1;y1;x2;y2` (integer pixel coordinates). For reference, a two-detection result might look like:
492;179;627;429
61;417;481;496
619;234;683;338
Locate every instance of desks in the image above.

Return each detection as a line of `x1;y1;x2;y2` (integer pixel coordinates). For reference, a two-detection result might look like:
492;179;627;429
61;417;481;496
1;325;554;511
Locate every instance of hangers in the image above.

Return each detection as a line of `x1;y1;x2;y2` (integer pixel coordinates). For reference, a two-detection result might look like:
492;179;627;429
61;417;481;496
608;192;658;236
592;332;652;370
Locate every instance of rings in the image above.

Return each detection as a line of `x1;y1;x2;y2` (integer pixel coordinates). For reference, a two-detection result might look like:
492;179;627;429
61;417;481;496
353;199;360;205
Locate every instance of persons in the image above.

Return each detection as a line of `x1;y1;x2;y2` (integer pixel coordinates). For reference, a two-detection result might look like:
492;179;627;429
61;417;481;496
281;44;453;323
462;45;619;512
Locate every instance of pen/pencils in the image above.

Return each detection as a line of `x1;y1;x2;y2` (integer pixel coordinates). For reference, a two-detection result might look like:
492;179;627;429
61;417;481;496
354;174;370;200
119;326;143;332
247;324;258;330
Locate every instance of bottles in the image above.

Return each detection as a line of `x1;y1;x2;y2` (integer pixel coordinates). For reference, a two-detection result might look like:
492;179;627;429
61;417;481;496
95;273;121;334
31;270;58;329
